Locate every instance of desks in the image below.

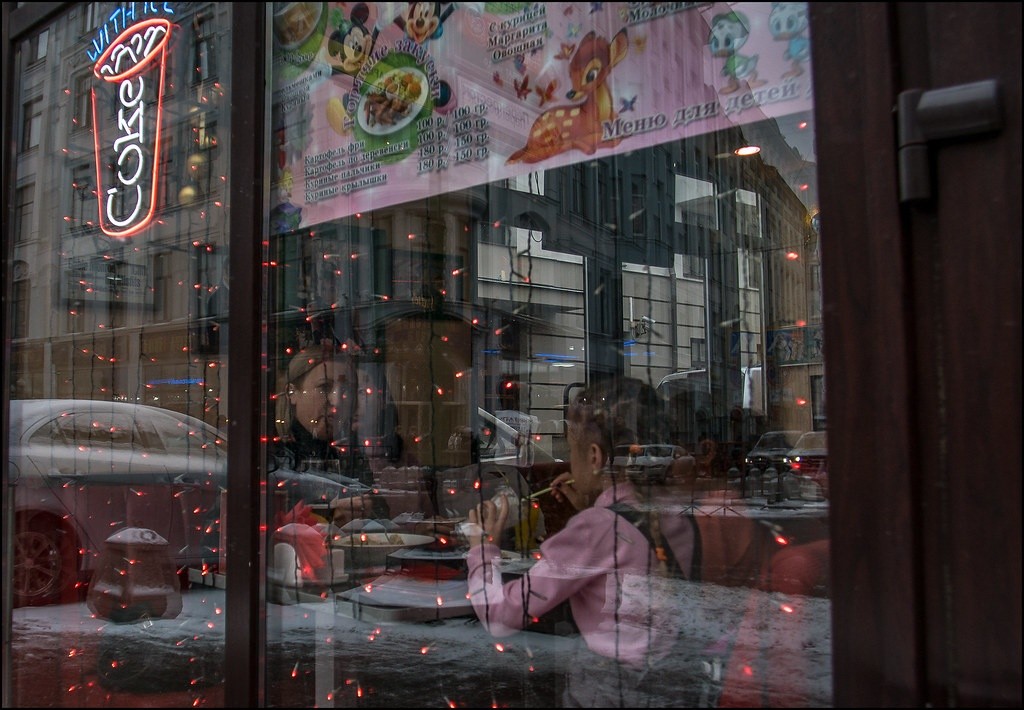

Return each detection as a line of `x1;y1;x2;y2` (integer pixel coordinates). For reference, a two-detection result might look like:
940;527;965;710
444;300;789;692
187;563;512;626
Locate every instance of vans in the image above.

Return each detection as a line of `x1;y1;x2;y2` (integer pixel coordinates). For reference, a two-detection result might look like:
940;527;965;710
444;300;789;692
654;367;762;415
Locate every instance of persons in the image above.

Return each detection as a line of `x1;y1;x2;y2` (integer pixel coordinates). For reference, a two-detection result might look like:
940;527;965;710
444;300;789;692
467;377;830;708
199;344;460;600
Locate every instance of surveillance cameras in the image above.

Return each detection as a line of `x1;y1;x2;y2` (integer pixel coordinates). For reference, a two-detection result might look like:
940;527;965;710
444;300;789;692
641;316;655;324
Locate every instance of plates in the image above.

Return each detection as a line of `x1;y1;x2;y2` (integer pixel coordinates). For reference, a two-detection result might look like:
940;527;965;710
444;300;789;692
274;2;322;49
357;67;428;135
333;533;435;555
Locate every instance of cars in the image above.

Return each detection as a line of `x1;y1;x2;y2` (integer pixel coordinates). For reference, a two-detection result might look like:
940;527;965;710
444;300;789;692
476;407;564;469
746;430;801;473
784;430;827;484
377;465;547;555
623;445;696;485
607;447;640;475
8;398;375;603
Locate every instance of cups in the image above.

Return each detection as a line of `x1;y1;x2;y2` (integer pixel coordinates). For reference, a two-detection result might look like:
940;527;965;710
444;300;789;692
455;487;528;549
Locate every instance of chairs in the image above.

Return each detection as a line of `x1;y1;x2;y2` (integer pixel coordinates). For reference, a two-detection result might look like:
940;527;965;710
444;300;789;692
434;462;546;538
374;464;438;519
